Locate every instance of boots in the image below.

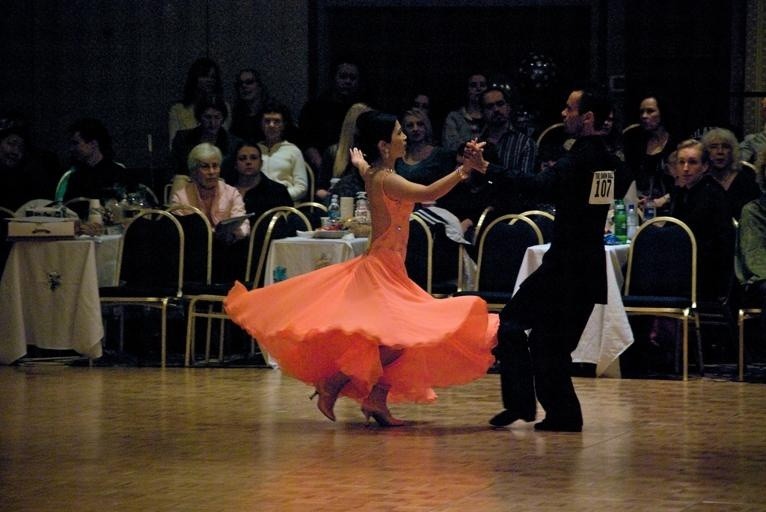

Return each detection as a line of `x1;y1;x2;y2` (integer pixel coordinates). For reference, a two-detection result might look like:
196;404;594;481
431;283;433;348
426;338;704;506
308;345;406;425
361;380;411;430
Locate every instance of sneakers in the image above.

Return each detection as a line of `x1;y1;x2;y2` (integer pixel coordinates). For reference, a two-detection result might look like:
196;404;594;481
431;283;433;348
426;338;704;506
489;405;536;426
535;419;583;434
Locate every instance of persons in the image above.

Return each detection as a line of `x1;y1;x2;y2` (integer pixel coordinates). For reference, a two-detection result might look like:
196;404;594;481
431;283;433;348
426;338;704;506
463;83;619;431
222;109;486;427
0;58;764;379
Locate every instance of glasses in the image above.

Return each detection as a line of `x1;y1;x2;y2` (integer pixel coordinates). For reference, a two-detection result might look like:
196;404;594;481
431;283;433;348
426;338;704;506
237;78;258;87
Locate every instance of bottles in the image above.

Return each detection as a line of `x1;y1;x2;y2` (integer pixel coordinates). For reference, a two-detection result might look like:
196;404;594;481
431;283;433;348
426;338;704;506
104;191;152;235
325;191;367;221
612;197;657;245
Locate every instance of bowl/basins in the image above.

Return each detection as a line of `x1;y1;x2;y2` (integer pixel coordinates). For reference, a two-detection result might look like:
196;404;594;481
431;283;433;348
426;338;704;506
295;230;313;240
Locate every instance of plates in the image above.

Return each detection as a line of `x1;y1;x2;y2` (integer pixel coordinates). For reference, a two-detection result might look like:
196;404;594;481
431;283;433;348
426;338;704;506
314;231;344;239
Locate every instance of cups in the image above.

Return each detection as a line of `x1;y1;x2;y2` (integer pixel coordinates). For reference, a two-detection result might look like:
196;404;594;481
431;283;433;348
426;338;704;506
87;198;102;225
320;216;330;227
